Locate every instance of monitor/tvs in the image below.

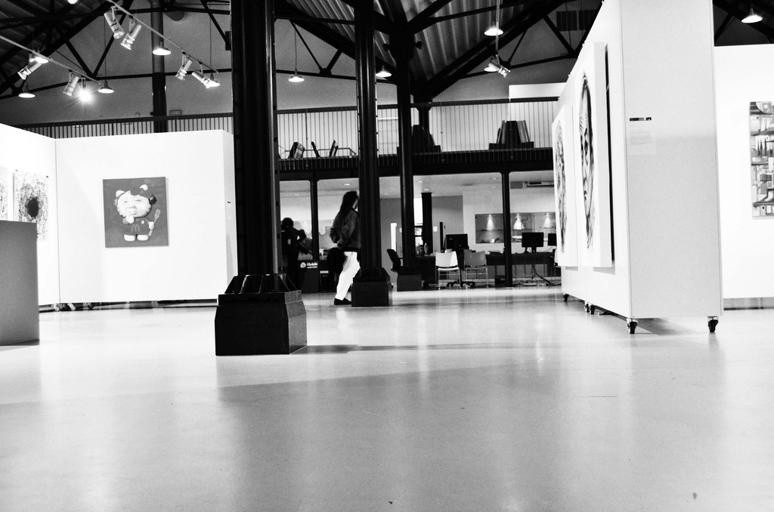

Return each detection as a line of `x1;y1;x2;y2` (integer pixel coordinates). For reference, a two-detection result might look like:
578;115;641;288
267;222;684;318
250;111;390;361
521;232;544;253
446;234;468;252
548;233;557;246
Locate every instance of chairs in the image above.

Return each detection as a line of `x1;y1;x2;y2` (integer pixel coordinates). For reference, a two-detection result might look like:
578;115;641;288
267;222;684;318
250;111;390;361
387;249;415;273
464;249;488;288
435;251;461;290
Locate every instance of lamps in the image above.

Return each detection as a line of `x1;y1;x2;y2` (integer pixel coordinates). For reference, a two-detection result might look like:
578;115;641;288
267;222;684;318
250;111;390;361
482;18;511;80
373;62;396;81
104;4;145;50
95;19;116;98
149;39;223;91
286;31;306;85
16;46;87;99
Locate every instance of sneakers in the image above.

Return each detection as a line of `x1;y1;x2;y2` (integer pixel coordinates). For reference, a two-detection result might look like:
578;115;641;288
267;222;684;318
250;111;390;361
334;298;351;305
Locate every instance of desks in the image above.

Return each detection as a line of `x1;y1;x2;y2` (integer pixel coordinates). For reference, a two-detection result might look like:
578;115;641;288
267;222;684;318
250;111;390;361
486;252;554;287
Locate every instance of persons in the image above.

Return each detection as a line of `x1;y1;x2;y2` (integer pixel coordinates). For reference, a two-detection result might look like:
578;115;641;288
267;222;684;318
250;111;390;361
281;218;312;280
554;120;565;246
579;79;594;248
330;191;361;305
